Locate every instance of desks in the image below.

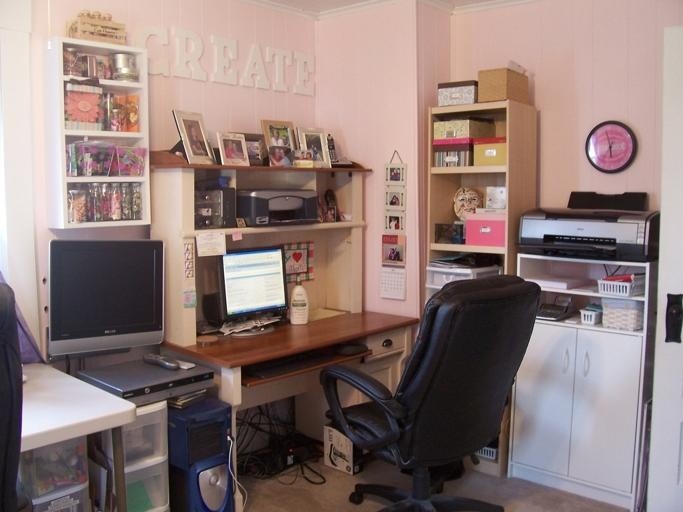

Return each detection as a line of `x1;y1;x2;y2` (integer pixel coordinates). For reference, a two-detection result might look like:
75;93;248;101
14;359;137;512
163;307;423;512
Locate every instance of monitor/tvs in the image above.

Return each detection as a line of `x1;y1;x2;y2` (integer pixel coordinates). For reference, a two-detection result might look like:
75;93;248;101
202;244;289;337
48;239;164;357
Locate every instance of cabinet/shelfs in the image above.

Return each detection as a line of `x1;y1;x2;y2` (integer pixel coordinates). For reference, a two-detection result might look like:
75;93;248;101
506;252;652;510
425;100;539;479
46;38;156;229
150;156;375;347
15;435;97;512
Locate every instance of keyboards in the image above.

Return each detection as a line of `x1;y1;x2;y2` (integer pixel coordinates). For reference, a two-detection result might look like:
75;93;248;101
243;347;336;379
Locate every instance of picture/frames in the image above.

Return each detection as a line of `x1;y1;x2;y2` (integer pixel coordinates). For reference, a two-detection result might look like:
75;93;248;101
259;118;297;167
166;109;218;163
215;131;251;166
278;239;314;284
238;132;269;166
294;125;333;168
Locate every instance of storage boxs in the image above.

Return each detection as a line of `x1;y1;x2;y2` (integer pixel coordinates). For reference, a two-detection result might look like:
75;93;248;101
432;144;473;166
465;212;506;247
472;136;506;166
433;118;496;146
436;79;477;106
475;67;531;102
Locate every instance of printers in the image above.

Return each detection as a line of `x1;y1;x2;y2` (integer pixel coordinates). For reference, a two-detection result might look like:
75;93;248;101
514;191;660;262
237;188;318;226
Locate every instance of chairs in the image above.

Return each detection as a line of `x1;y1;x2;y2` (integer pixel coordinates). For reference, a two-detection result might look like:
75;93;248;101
319;272;543;511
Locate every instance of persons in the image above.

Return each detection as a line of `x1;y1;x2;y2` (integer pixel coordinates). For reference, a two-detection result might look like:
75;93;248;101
382;161;405;263
185;122;343;170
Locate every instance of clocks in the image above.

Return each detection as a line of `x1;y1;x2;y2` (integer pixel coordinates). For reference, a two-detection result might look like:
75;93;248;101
584;119;638;174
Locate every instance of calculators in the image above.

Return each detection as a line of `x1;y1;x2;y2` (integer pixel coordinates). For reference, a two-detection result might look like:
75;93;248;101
535;303;568;321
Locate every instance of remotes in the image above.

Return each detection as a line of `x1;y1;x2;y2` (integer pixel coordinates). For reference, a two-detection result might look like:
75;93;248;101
324;189;342;222
143;353;179;370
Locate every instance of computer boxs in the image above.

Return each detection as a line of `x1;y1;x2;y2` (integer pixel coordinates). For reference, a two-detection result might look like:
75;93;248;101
168;397;235;512
195;183;236;230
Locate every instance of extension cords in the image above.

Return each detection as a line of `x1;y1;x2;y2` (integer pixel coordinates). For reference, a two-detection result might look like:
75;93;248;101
258;451;301;476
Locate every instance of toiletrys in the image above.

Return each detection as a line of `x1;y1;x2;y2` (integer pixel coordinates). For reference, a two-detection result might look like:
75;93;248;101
289;276;308;324
